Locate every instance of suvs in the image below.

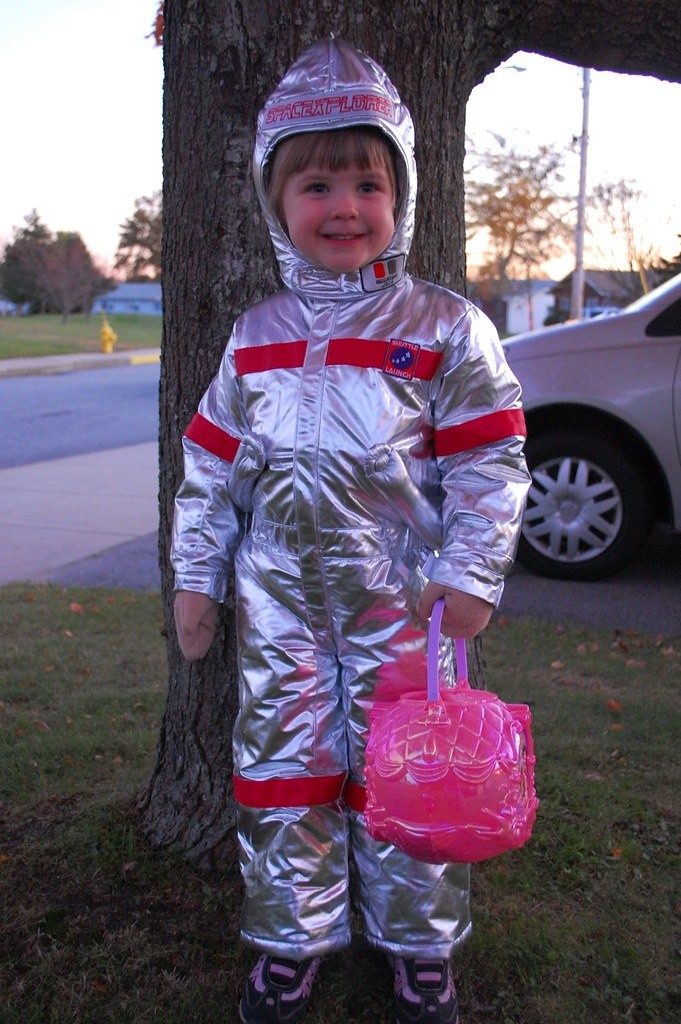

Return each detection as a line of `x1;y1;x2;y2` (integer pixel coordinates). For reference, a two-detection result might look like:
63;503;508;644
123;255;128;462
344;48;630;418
498;271;681;584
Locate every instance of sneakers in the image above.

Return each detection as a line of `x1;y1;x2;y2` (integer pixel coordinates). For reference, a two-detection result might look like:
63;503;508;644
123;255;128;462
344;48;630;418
239;952;326;1024
391;955;460;1024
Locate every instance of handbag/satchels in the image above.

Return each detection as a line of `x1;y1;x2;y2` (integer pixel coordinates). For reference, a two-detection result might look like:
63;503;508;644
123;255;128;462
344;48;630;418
363;597;540;865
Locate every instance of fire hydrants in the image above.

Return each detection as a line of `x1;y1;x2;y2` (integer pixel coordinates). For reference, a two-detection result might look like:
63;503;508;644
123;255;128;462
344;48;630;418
98;320;116;353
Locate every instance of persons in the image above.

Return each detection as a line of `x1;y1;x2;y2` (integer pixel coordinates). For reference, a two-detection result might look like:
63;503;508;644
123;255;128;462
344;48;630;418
169;30;533;1023
